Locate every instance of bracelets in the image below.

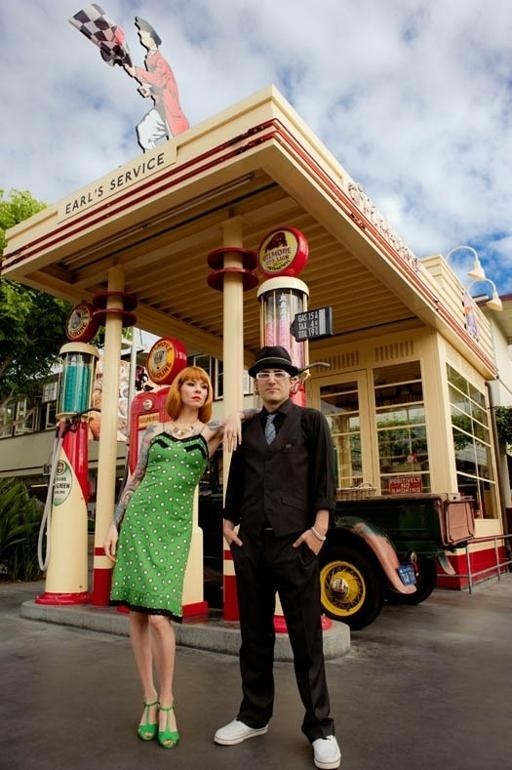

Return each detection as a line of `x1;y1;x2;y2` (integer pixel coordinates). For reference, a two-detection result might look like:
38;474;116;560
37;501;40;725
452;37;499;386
311;527;326;542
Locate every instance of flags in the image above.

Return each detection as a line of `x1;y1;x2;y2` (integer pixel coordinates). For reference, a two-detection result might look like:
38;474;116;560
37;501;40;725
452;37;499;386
70;2;134;70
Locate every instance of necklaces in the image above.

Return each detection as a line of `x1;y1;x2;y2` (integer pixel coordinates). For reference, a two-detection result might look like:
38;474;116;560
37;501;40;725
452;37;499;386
170;420;200;436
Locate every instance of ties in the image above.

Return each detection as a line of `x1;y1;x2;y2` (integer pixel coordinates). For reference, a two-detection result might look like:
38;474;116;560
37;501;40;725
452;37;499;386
265;413;280;447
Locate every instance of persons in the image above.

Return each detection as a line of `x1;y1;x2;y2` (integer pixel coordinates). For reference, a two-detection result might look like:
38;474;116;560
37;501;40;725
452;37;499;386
211;344;342;769
102;362;261;748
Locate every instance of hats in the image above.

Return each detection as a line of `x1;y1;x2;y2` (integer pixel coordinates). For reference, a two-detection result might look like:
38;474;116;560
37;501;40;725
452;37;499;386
248;346;298;377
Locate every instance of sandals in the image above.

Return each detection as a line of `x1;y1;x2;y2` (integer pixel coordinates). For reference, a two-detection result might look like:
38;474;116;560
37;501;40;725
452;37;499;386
137;700;181;749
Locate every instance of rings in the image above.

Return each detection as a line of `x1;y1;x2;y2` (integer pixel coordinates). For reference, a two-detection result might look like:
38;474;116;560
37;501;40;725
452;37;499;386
232;435;237;437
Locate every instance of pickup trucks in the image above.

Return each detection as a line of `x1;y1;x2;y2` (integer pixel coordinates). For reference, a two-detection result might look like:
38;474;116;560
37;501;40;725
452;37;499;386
199;444;481;633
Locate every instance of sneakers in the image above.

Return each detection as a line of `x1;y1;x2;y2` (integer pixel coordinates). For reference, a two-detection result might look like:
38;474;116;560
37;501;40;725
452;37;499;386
214;716;269;745
311;735;341;769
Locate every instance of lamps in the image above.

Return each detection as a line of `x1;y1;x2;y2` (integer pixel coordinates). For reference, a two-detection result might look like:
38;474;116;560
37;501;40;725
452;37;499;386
446;246;486;280
468;278;503;312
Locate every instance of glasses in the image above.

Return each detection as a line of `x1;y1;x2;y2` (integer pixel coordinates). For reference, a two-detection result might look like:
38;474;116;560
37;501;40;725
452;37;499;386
256;372;292;380
